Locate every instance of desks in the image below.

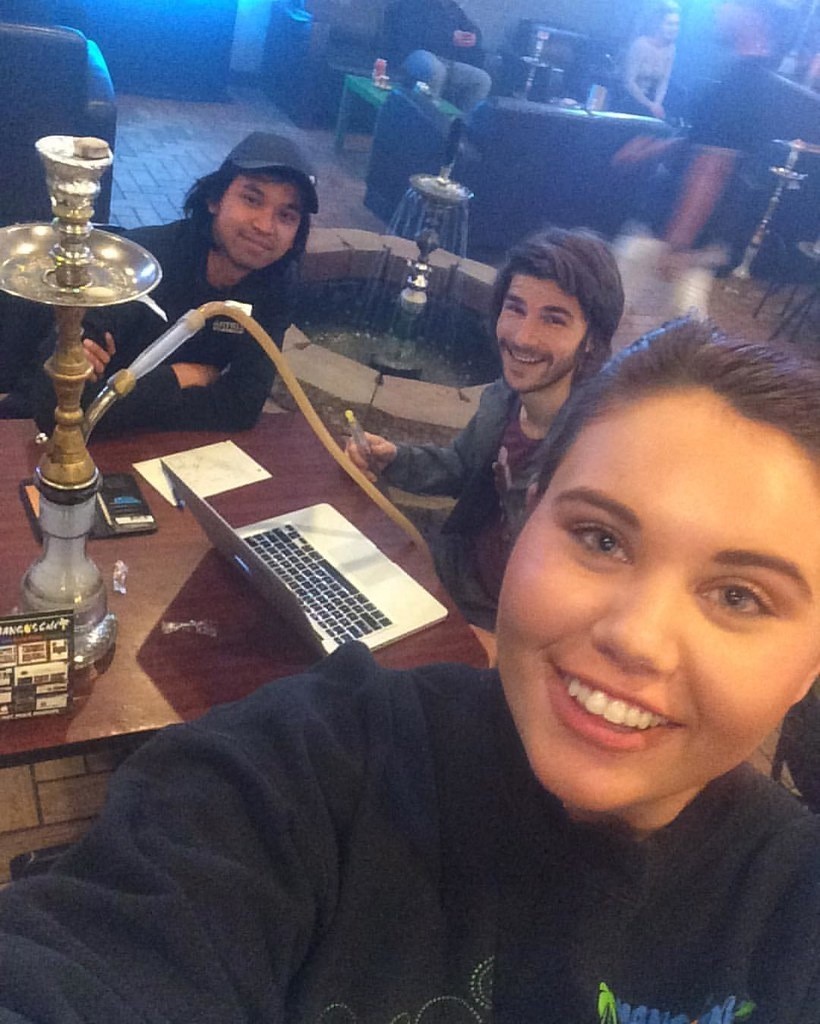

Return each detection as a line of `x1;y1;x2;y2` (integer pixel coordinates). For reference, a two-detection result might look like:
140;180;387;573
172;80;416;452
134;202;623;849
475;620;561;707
1;413;490;767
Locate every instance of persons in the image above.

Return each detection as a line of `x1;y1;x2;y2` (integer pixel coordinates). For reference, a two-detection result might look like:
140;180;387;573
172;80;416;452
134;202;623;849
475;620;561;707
608;0;683;166
394;0;491;112
344;225;624;629
0;313;820;1024
1;131;318;433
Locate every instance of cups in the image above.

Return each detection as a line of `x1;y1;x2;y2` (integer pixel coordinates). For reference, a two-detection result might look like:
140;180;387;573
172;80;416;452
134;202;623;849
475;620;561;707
590;84;607;112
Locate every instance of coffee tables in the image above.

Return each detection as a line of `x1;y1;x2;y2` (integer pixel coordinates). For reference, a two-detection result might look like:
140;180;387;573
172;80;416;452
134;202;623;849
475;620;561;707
338;75;464;162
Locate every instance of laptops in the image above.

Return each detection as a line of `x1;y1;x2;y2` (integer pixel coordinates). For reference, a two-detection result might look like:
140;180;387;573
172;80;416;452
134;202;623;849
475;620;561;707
160;458;448;659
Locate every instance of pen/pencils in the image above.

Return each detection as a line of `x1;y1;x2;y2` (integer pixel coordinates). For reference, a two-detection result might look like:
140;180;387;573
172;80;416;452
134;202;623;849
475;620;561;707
345;409;389;495
93;493;115;529
170;483;186;510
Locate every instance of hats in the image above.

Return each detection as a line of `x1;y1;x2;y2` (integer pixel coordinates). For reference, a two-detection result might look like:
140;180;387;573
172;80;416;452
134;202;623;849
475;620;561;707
228;131;320;215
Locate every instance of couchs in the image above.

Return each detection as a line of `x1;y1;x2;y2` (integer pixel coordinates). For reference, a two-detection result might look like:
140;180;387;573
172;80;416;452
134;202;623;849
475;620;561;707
0;22;117;393
265;0;674;258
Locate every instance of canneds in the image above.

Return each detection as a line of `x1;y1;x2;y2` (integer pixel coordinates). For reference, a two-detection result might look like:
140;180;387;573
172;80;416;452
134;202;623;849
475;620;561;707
372;59;387;83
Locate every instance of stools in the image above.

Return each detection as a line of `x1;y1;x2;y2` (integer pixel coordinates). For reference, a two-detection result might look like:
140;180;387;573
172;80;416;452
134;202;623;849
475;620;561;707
754;240;820;342
